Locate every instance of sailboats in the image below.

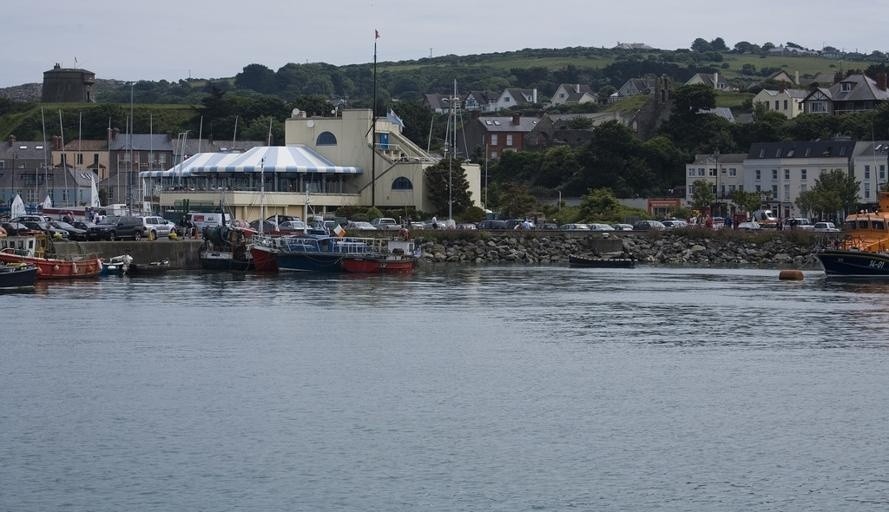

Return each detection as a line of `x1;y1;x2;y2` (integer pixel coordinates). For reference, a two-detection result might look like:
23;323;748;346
0;82;273;224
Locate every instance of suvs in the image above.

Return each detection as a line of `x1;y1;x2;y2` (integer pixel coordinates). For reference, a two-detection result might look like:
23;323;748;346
86;216;145;241
137;216;178;241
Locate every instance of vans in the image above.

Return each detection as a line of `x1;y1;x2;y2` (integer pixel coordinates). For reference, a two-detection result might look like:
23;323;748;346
184;212;231;233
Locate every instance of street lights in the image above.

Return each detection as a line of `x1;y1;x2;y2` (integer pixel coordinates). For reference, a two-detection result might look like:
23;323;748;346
712;147;720;205
441;95;459;221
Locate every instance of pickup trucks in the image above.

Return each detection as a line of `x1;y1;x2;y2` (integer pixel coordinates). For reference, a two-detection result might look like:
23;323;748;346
370;217;402;231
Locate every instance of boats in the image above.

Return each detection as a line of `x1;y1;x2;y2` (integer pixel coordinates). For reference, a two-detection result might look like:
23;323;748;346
0;282;37;297
0;231;103;278
101;254;171;278
814;189;889;279
0;261;39;282
568;252;635;268
198;158;425;275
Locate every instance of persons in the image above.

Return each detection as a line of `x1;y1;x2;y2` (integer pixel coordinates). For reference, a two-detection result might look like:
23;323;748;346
399;225;410;241
513;219;535;230
187;219;193;239
180;215;188;239
790;216;798;231
776;217;783;231
724;213;732;227
431;214;439;229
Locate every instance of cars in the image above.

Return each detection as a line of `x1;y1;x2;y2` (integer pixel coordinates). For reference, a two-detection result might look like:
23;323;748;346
411;210;840;232
265;215;300;225
313;220;336;230
0;214;90;241
248;219;278;235
278;220;311;230
343;222;377;230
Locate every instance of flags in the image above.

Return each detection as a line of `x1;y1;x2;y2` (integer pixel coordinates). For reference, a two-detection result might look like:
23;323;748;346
332;221;347;239
